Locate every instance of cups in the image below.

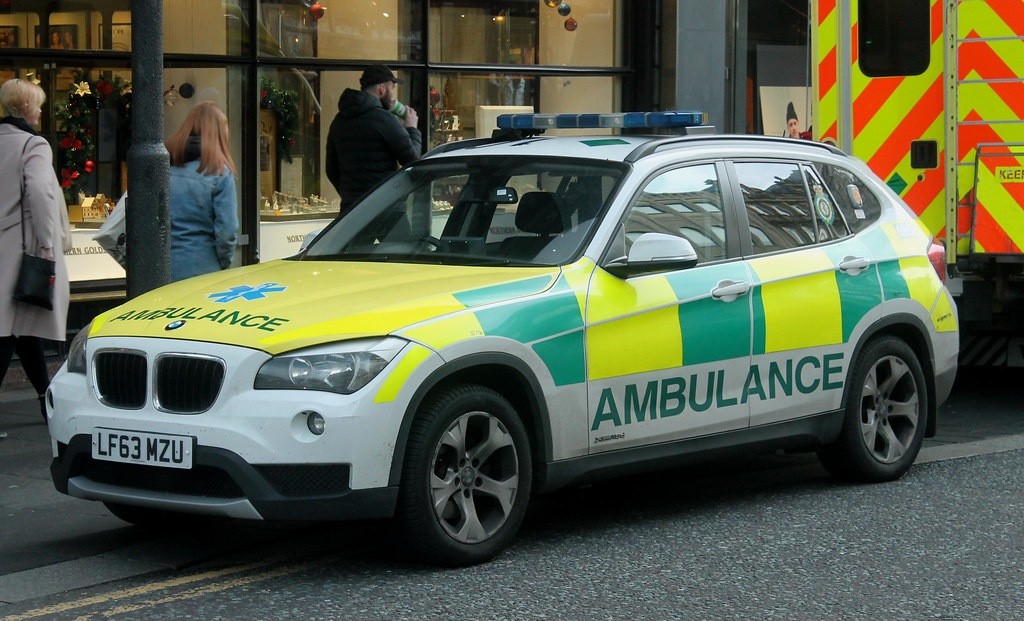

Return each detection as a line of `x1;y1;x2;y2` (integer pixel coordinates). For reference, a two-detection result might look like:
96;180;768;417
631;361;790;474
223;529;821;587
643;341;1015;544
389;100;407;120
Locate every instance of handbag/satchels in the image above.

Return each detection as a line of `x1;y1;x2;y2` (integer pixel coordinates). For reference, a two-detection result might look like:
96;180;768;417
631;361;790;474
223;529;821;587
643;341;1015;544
12;251;55;311
92;188;128;270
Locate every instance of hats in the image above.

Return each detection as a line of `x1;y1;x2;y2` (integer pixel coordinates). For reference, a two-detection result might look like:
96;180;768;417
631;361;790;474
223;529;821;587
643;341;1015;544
360;65;403;88
787;102;798;124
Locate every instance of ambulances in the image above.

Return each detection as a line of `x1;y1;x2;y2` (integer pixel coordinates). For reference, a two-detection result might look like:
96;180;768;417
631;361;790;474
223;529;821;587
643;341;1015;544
45;110;961;563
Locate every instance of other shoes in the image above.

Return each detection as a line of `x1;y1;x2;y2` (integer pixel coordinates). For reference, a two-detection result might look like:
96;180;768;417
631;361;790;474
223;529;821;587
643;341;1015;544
38;394;47;422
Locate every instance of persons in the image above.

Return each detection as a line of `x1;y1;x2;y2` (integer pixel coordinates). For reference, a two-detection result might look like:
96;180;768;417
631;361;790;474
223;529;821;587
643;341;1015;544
325;66;422;252
503;74;530;106
0;78;73;438
786;102;811;140
163;101;239;282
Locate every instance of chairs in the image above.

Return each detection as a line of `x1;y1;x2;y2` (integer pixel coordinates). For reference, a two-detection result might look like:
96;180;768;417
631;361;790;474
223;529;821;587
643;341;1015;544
495;192;577;261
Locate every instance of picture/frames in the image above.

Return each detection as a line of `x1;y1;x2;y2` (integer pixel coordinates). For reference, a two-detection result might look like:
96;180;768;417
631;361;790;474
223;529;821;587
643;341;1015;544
34;24;80;93
0;25;20;88
98;22;132;81
